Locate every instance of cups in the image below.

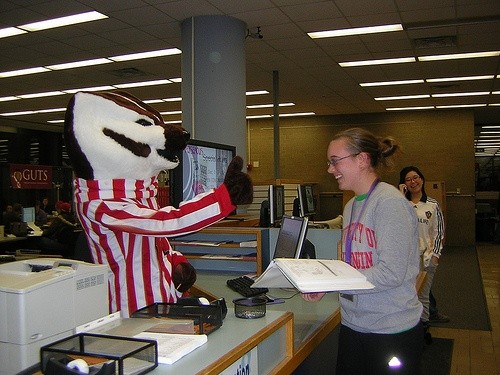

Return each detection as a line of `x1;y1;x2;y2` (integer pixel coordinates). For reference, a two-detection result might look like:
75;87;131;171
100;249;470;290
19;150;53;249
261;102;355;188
457;188;460;194
0;226;4;239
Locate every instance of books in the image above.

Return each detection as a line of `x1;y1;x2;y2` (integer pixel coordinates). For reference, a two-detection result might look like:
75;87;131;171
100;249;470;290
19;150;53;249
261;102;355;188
251;258;374;293
133;332;207;364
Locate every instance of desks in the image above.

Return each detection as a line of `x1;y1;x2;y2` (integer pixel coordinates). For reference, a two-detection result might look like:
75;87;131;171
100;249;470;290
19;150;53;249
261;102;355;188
3;224;343;375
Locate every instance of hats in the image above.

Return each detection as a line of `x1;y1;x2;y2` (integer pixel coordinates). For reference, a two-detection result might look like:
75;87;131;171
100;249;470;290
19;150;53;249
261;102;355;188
59;202;70;212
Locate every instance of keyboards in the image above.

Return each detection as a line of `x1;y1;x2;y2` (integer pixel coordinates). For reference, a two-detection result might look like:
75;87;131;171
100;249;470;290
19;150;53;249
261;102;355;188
227;275;268;298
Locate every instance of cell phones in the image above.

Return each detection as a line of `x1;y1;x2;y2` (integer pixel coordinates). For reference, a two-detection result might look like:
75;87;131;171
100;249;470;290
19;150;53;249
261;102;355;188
404;187;407;192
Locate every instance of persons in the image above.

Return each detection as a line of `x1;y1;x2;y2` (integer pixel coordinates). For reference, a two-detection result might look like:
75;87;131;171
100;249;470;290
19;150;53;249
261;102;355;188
0;197;78;258
398;166;449;331
301;127;424;375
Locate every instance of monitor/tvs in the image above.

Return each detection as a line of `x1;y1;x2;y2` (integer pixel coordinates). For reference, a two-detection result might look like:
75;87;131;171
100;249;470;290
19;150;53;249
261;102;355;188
273;215;308;260
297;184;316;215
169;137;244;220
269;184;285;226
23;207;36;224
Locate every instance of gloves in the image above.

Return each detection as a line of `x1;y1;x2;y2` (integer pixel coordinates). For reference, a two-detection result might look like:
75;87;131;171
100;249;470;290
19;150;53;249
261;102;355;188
172;261;196;292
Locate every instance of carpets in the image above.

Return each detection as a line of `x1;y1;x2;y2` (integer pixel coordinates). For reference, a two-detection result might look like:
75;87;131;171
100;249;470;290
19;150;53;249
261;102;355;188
427;244;494;332
414;336;455;375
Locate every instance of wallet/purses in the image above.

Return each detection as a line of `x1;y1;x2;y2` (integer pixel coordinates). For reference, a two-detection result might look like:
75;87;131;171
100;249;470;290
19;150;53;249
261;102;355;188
223;155;254;206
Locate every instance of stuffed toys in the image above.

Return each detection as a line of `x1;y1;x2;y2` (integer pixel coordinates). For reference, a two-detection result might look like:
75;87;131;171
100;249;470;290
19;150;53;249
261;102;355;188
64;89;254;317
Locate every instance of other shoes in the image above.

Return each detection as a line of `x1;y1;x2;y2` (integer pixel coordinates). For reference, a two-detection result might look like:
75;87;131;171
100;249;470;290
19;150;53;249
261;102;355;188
429;311;450;323
423;321;432;345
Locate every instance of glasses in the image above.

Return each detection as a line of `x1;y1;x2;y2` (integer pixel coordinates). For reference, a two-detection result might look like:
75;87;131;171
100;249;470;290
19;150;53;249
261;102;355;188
407;175;421;182
327;152;361;166
